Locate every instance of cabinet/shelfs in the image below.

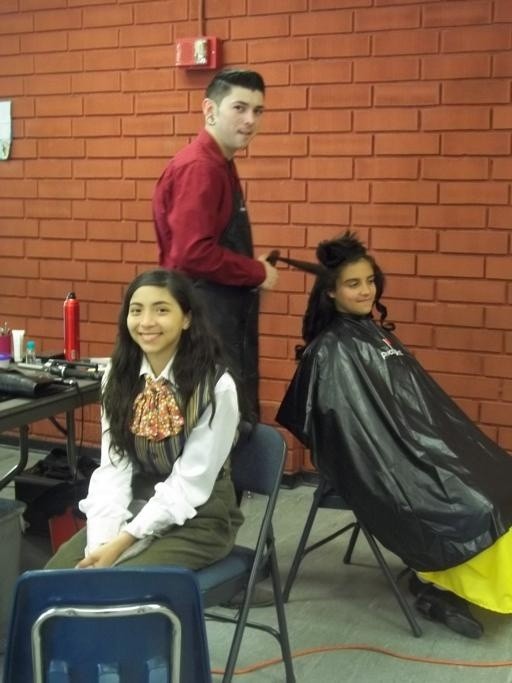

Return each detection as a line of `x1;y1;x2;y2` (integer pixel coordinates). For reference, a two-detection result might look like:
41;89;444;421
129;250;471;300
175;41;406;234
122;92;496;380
63;292;80;361
26;340;36;364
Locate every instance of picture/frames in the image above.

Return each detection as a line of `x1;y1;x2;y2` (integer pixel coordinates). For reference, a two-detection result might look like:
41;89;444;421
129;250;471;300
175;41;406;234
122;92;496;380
0;368;100;492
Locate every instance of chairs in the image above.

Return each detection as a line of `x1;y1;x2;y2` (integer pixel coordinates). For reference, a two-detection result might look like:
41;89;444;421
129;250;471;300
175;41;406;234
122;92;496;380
220;585;275;609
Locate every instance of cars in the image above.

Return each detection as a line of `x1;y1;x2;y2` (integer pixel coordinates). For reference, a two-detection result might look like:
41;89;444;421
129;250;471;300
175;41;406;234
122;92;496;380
0;332;11;369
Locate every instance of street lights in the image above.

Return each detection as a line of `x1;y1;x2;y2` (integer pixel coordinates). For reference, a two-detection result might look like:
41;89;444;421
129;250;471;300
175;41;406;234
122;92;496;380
0;366;78;398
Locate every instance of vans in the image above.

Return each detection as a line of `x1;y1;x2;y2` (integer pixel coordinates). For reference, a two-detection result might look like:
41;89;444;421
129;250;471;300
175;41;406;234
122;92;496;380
407;571;431;597
413;583;484;640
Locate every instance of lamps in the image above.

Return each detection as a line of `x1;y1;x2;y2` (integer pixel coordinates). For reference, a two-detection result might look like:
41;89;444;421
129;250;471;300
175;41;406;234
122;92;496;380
191;423;296;682
3;565;212;682
282;344;422;638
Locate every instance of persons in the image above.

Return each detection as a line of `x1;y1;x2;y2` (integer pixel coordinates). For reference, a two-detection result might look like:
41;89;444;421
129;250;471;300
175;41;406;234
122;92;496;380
42;268;245;574
274;230;512;639
152;67;277;609
